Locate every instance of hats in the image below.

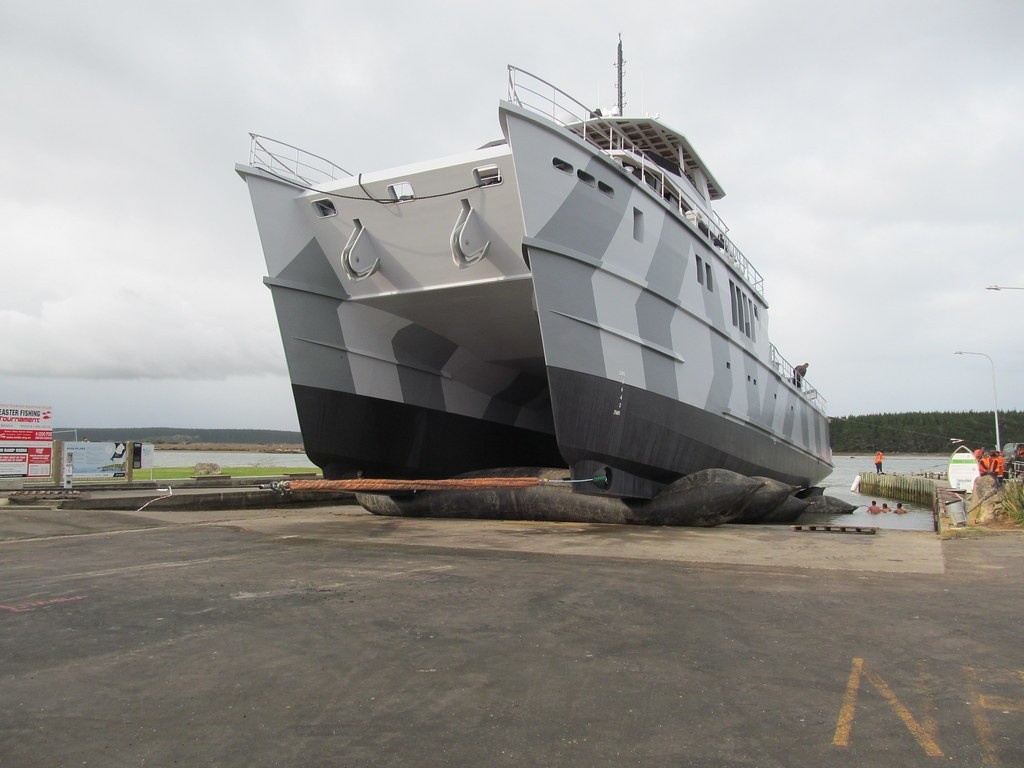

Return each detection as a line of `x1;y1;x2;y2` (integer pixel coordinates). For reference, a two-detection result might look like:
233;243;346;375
989;450;1000;456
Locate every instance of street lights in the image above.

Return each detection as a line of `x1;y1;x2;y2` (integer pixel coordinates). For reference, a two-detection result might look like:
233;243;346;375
953;351;1002;454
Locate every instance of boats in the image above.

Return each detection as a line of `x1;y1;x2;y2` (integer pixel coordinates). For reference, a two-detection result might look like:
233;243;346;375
234;33;836;503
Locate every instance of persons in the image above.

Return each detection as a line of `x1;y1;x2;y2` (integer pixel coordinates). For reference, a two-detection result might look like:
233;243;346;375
792;363;809;389
874;450;883;474
974;445;1024;487
866;500;907;514
